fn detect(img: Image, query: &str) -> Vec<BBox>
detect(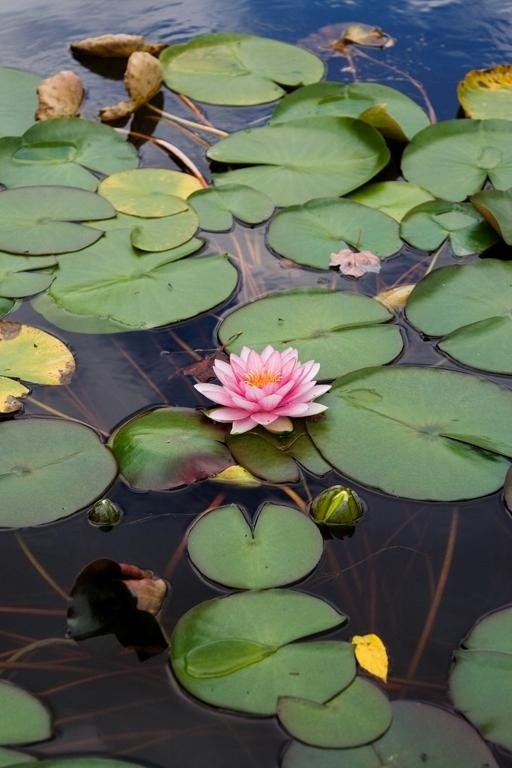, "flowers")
[194,344,333,442]
